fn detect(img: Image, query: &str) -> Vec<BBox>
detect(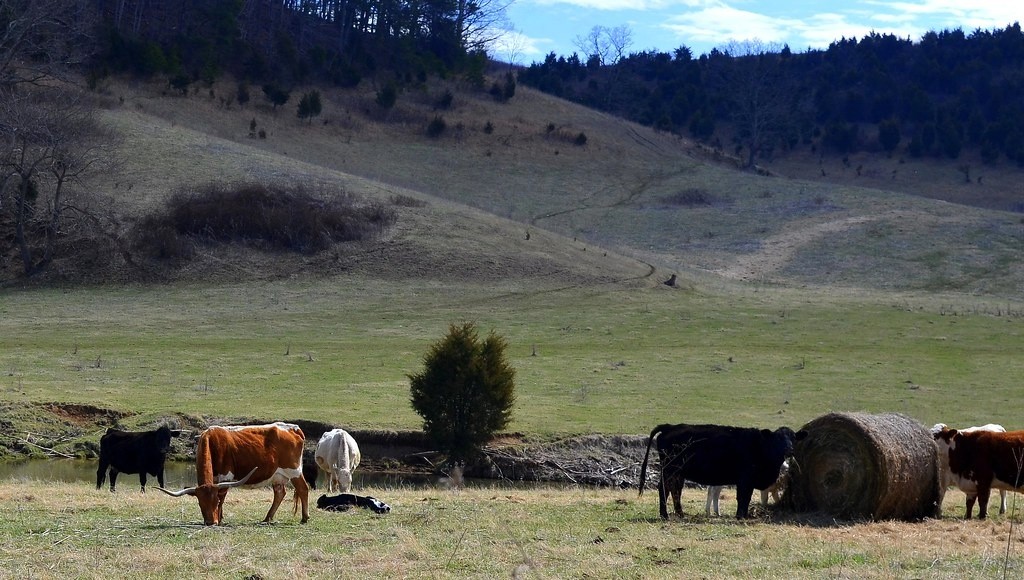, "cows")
[637,424,808,522]
[928,422,1024,520]
[151,422,309,526]
[96,421,180,494]
[302,428,391,514]
